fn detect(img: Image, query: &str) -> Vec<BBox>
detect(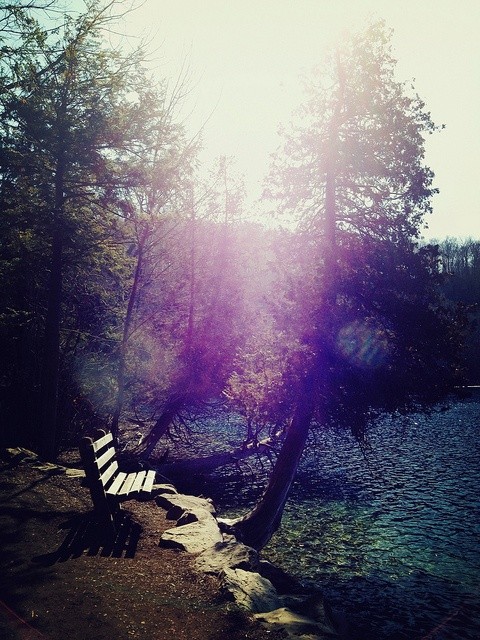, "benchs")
[80,429,155,533]
